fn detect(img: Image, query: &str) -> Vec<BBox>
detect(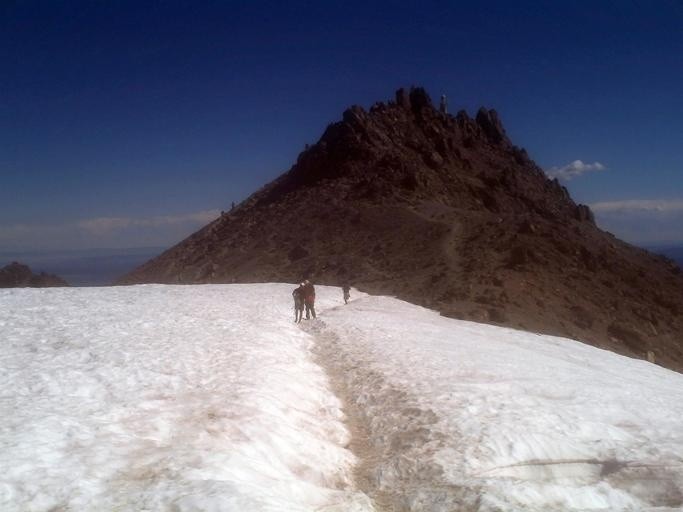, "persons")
[291,283,305,323]
[303,279,317,320]
[342,281,351,305]
